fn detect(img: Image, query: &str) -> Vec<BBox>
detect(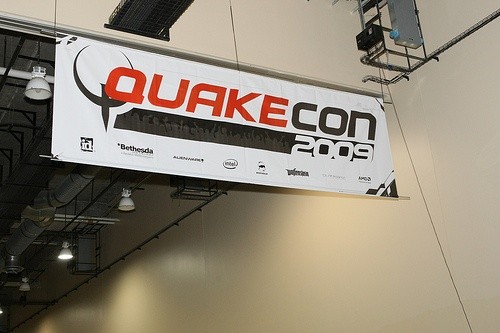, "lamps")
[0,306,3,314]
[24,36,52,100]
[117,169,136,210]
[57,206,74,260]
[18,250,30,291]
